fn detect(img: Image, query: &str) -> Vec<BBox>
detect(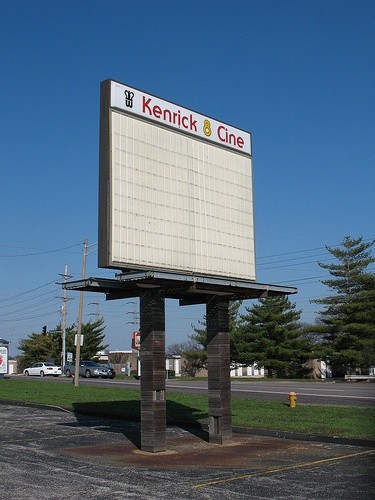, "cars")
[65,362,111,378]
[22,362,62,378]
